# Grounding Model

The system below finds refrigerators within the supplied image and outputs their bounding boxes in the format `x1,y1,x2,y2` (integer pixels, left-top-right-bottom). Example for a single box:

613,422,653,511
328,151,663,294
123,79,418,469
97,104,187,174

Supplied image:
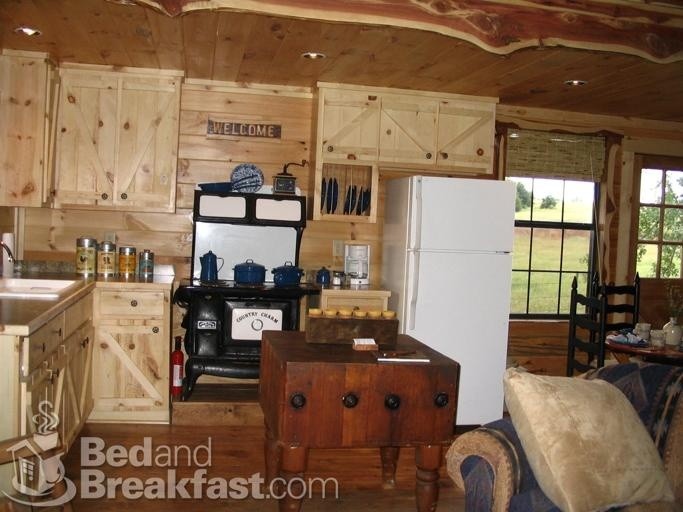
381,174,518,429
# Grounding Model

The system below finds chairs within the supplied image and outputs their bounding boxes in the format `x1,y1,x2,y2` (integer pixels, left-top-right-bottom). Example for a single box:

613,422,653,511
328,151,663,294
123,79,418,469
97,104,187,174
589,271,641,360
566,277,608,375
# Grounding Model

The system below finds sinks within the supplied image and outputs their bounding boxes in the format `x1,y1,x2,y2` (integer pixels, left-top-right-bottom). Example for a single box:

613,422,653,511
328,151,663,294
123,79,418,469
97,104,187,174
0,278,83,299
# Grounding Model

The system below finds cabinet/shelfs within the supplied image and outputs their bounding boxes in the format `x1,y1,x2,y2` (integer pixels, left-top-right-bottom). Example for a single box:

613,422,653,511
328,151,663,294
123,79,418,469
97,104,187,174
308,79,500,221
53,57,183,215
88,278,172,427
0,294,94,484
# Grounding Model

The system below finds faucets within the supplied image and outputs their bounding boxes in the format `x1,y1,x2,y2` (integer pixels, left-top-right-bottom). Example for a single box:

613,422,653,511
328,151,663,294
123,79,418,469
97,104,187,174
0,241,15,264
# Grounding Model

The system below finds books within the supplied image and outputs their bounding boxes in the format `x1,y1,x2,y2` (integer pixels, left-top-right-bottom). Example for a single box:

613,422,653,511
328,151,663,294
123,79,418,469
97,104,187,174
368,348,431,363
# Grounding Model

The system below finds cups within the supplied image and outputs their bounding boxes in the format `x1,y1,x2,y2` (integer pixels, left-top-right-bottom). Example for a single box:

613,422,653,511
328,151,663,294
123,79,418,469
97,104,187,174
649,330,667,348
634,322,652,339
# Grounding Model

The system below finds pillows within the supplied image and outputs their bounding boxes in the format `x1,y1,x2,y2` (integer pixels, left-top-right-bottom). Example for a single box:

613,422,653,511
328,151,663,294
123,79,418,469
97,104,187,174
504,366,674,512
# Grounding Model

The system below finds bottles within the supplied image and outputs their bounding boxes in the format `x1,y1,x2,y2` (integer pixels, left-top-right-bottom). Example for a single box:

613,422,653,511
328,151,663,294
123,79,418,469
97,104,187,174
663,316,681,346
74,237,96,282
117,246,135,283
168,335,183,397
96,241,115,282
139,248,153,283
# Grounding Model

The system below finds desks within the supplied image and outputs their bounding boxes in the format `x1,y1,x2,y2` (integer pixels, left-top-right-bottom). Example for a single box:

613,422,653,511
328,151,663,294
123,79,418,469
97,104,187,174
259,329,460,512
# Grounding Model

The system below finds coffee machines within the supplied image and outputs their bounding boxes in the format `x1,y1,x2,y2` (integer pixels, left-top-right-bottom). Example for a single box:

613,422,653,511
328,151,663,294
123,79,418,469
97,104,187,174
343,238,370,285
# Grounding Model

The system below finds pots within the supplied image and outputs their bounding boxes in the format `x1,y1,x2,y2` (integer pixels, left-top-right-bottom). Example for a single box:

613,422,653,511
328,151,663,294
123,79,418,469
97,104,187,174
197,183,233,194
272,260,305,286
232,258,266,286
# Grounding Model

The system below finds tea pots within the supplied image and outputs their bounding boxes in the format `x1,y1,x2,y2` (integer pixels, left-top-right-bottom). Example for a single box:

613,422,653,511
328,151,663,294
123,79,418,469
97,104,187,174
316,266,330,284
199,249,224,287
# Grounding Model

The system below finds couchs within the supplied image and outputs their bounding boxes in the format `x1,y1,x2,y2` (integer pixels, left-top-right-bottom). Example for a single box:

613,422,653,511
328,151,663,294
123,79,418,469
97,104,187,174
447,361,683,512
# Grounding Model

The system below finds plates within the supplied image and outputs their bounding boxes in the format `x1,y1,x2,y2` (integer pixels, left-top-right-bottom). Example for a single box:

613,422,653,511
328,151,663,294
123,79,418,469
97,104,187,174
229,164,264,194
317,177,370,215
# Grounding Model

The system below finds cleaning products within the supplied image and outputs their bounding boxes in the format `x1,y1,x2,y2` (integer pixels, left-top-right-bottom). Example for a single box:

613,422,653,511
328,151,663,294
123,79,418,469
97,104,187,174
2,232,14,265
